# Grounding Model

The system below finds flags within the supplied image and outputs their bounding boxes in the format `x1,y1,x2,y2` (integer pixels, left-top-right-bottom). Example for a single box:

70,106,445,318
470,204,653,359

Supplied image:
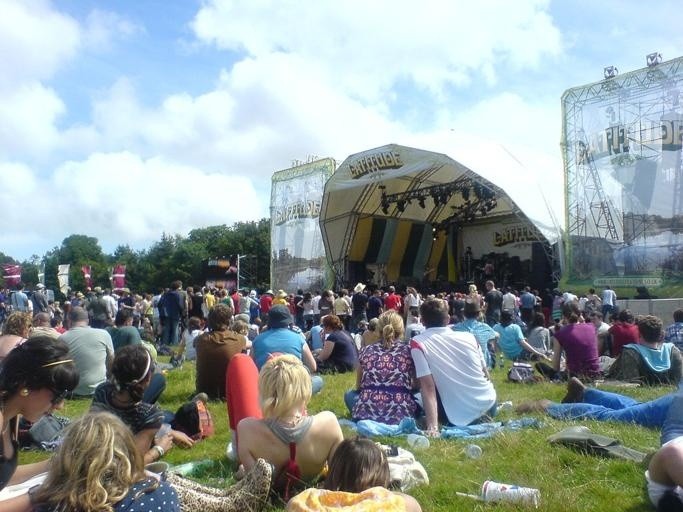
37,263,125,295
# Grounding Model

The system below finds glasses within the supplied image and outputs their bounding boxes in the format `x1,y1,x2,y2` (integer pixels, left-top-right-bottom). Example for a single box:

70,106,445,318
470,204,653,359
49,386,72,404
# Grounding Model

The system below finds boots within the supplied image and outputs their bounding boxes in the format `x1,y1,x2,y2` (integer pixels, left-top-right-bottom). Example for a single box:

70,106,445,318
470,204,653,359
160,458,274,512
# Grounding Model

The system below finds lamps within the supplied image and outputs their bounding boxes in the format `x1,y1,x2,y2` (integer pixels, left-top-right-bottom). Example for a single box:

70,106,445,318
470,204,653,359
430,194,499,241
646,52,663,66
378,175,498,216
602,65,619,79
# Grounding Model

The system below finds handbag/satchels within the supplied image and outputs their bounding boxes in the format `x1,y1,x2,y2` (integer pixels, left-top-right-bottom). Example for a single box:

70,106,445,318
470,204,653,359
377,439,429,491
510,363,532,381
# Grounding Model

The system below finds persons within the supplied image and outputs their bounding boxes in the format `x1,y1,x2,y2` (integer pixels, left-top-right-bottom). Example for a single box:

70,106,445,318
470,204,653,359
482,261,494,279
0,280,683,512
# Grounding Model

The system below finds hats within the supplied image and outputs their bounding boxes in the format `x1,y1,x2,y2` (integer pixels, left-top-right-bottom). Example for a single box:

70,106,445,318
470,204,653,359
354,283,365,293
268,305,292,326
266,290,273,294
277,290,287,298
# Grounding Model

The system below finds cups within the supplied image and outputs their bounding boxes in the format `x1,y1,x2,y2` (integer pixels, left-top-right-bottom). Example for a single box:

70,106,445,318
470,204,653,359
144,460,169,475
405,432,430,453
480,480,540,509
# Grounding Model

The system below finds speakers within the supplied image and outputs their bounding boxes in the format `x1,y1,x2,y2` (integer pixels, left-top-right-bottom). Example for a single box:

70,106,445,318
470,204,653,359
532,242,552,290
348,260,366,291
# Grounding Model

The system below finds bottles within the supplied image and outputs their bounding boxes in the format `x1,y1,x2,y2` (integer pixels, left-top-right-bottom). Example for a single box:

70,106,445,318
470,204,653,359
465,442,483,459
152,423,171,443
